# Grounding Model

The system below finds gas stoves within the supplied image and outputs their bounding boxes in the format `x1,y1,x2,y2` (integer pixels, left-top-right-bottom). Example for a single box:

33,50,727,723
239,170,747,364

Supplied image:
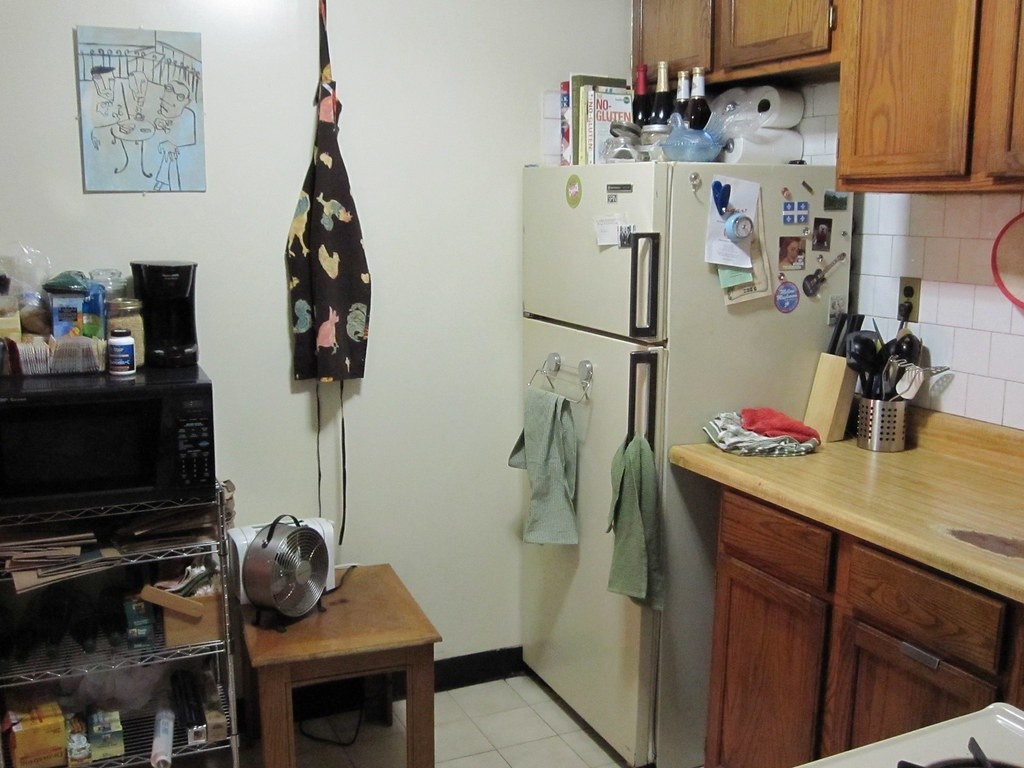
793,701,1024,768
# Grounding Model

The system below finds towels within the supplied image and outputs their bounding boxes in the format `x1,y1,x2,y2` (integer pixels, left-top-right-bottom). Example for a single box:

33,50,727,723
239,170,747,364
505,384,579,546
606,432,663,604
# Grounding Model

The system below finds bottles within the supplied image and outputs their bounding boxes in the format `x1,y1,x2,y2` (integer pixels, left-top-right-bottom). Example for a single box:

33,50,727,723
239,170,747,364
641,125,670,145
631,60,711,131
107,330,136,375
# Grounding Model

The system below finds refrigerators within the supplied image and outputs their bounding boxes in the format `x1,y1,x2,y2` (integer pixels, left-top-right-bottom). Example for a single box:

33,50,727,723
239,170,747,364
519,162,854,768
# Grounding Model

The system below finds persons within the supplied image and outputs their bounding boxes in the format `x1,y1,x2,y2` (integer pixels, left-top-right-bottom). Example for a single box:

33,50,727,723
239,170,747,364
780,237,802,269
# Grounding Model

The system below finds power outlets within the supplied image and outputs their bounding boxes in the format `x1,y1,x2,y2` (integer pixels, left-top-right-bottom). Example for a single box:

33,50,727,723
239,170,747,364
896,276,923,322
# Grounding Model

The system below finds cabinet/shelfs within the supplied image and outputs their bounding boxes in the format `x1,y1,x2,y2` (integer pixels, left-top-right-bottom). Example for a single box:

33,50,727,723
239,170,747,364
0,480,248,768
633,0,1024,195
698,487,1024,768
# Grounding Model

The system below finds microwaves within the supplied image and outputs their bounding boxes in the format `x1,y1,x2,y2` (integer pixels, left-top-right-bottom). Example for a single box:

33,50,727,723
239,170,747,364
0,362,217,516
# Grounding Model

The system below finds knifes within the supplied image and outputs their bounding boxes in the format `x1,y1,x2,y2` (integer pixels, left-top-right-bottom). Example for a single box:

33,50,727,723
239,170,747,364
827,313,865,356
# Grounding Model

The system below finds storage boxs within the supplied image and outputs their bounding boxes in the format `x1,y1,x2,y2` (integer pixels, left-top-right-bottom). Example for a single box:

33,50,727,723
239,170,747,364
165,592,223,646
7,693,68,768
87,709,126,761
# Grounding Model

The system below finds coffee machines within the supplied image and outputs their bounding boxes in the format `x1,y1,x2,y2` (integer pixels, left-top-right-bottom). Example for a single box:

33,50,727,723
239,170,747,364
129,260,199,368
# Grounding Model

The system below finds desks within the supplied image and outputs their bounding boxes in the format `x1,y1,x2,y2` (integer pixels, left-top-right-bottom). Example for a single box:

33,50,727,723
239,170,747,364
240,561,445,768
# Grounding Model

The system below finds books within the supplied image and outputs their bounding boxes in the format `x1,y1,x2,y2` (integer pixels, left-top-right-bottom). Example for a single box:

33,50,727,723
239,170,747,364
1,505,218,594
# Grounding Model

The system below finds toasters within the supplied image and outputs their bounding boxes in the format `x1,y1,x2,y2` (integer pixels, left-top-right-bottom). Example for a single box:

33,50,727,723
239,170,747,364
227,517,335,607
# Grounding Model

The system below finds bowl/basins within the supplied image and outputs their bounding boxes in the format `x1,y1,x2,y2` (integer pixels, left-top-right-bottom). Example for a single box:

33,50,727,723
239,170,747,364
661,142,722,162
